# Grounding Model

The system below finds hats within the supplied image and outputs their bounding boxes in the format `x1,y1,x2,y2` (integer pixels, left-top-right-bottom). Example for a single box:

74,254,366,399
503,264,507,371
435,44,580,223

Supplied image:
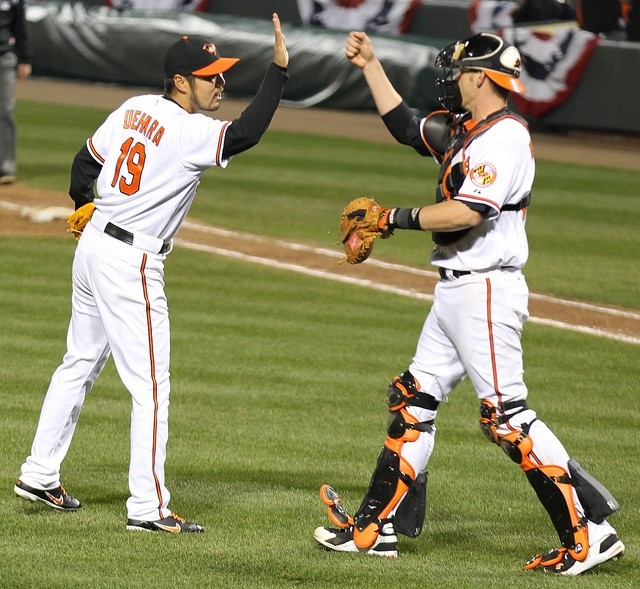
165,35,239,77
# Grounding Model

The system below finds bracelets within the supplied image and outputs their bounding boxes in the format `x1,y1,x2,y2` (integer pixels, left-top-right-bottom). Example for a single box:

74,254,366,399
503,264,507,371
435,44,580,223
388,207,424,231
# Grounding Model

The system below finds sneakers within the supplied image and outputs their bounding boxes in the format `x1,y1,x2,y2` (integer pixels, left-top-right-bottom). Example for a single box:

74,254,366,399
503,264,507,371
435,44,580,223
126,513,204,533
314,520,397,559
14,478,82,511
0,170,17,184
542,520,624,575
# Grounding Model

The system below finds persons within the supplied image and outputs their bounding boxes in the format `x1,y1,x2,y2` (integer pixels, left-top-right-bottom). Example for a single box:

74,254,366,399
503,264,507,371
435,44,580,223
312,32,625,577
15,13,290,536
1,1,31,185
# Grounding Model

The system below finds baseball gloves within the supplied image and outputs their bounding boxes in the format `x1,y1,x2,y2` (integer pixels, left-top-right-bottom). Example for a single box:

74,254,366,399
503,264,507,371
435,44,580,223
339,196,398,267
65,201,97,238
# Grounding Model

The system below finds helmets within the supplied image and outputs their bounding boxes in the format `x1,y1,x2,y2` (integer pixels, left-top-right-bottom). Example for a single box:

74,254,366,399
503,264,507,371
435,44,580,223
435,32,527,127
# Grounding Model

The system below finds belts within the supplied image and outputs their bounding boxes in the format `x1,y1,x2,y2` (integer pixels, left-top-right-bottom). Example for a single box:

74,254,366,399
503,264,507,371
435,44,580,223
89,209,172,255
438,266,515,280
